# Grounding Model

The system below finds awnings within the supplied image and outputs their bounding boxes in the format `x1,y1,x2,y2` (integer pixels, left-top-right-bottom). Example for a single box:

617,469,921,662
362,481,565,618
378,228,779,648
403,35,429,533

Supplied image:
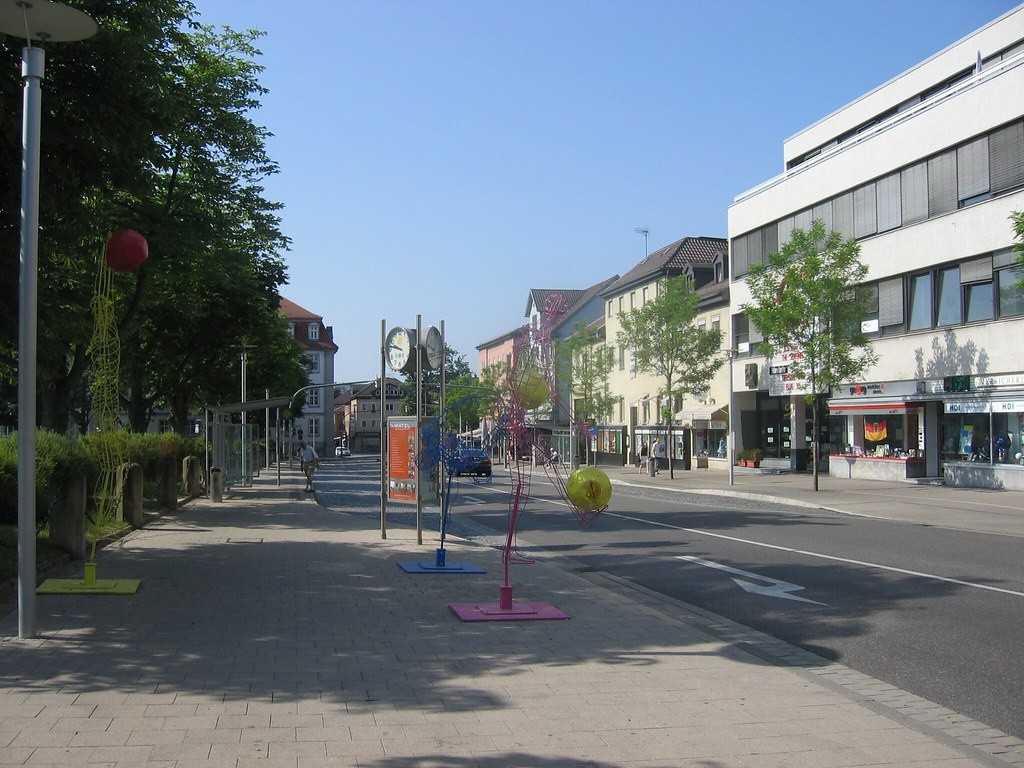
675,403,728,421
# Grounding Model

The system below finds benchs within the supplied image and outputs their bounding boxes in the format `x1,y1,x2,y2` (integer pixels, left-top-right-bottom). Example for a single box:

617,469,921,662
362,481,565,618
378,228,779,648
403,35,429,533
544,454,561,466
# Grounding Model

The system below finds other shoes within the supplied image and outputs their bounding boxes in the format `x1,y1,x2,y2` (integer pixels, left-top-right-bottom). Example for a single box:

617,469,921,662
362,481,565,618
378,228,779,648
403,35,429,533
303,489,314,493
546,465,551,469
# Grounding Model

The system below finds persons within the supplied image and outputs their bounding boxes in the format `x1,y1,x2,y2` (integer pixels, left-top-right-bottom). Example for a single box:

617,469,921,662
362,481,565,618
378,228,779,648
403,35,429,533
639,441,648,474
716,437,725,458
652,438,665,474
299,440,319,493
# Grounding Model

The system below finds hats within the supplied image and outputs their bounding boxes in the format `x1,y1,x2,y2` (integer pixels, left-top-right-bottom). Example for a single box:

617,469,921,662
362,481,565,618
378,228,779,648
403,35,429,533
550,448,554,451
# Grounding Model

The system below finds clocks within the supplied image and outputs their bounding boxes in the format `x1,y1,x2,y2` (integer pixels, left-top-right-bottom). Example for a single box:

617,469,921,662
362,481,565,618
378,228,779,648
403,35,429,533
422,325,443,372
385,327,417,372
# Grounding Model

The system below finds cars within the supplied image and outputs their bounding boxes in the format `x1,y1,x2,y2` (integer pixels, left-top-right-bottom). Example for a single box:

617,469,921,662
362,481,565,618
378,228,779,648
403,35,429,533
445,449,492,477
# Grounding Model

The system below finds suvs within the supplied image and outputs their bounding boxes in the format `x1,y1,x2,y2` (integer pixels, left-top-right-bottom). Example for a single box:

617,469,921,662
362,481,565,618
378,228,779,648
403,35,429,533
336,447,351,456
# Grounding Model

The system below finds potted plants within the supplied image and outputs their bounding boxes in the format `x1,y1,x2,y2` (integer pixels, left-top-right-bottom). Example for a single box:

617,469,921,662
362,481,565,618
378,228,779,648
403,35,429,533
735,449,765,468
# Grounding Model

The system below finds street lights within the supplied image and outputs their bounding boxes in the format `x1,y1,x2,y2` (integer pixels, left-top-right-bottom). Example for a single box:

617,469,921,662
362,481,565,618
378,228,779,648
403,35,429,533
265,385,269,473
232,344,258,486
2,3,97,638
714,349,744,486
